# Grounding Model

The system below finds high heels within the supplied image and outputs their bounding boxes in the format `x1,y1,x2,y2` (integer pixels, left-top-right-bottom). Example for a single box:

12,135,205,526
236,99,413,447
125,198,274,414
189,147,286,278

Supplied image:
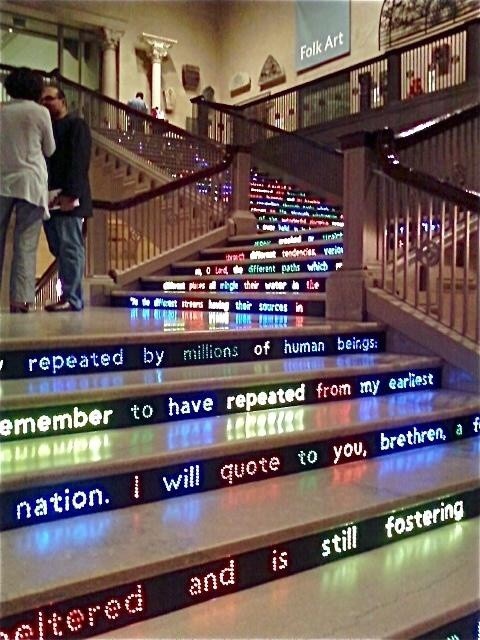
10,301,29,313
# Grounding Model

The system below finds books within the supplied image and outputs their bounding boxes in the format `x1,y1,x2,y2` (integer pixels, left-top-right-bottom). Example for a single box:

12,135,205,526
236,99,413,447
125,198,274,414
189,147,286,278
48,188,79,209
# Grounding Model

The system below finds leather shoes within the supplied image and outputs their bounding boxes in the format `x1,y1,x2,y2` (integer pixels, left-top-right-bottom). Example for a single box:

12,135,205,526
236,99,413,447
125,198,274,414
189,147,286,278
45,299,75,311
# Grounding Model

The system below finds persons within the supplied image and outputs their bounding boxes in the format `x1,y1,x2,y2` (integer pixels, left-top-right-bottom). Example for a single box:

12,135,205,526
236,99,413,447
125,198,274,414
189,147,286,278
40,86,93,311
126,92,148,133
274,113,282,131
1,66,55,313
